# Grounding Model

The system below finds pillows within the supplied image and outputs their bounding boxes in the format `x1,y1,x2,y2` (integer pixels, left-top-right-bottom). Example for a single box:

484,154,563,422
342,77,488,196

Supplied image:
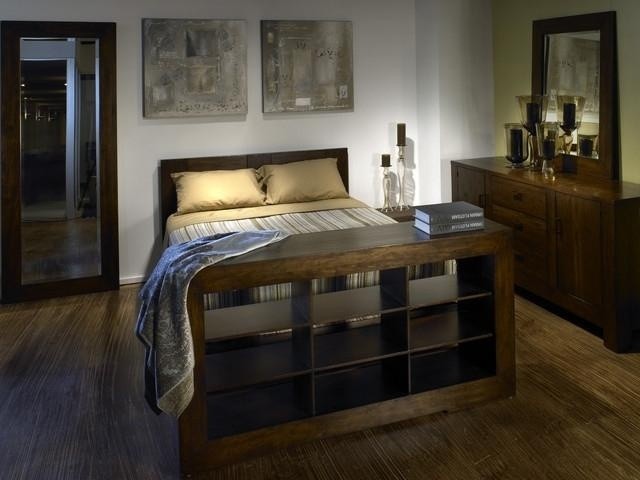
256,158,350,205
169,167,267,214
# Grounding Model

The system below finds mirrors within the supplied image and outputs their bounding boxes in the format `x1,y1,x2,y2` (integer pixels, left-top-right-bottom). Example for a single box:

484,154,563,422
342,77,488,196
531,12,617,180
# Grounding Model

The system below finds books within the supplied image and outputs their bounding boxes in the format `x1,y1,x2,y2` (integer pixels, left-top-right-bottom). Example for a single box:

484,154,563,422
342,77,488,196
412,201,483,224
412,217,484,236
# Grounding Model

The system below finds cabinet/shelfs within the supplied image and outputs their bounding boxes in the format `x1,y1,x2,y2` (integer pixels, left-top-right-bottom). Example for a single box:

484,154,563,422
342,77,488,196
451,155,640,354
178,217,515,474
636,153,640,156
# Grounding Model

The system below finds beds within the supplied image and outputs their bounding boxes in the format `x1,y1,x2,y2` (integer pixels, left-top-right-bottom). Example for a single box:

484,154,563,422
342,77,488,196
159,147,457,336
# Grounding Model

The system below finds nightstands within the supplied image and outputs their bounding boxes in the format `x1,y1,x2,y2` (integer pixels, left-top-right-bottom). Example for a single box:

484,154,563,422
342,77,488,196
376,206,415,222
